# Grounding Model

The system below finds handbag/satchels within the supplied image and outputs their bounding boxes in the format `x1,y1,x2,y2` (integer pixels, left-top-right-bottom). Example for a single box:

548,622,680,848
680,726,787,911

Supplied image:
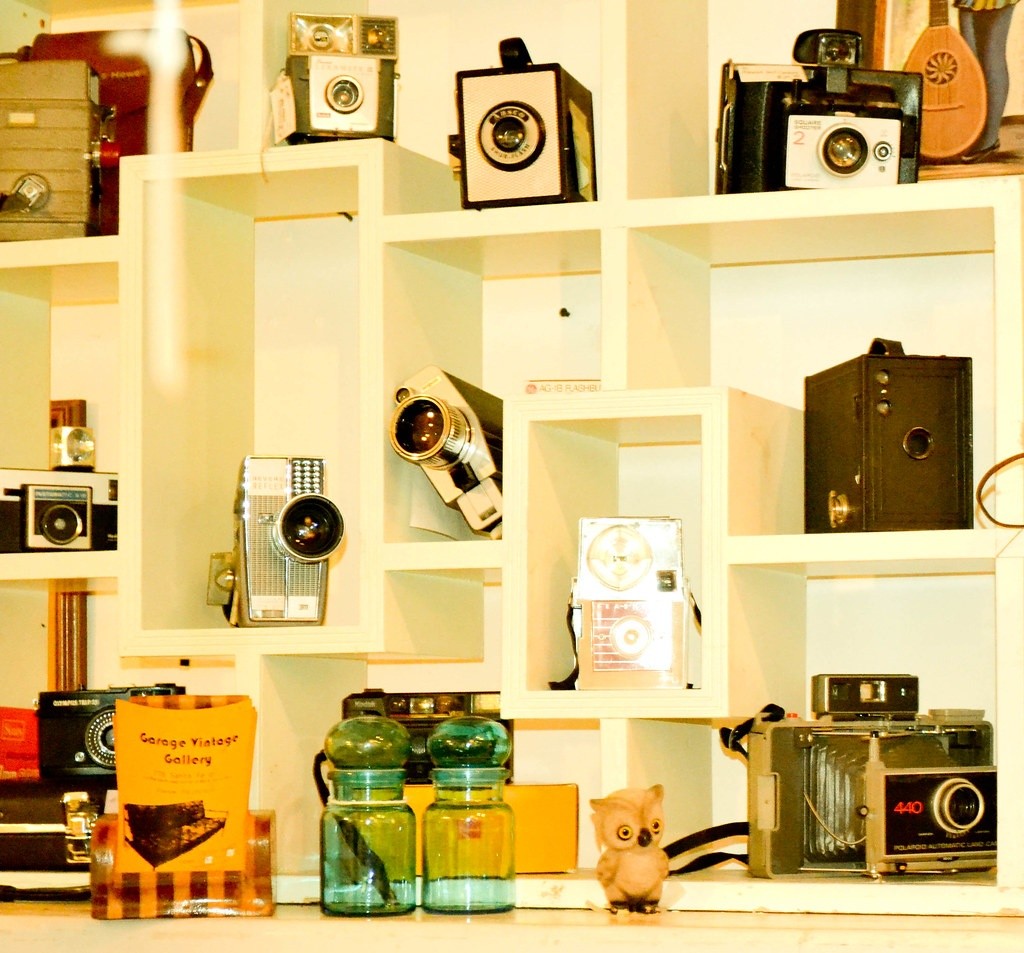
2,28,213,239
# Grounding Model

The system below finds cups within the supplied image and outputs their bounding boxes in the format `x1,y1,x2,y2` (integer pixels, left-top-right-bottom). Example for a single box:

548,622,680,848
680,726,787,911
421,716,517,915
317,716,416,913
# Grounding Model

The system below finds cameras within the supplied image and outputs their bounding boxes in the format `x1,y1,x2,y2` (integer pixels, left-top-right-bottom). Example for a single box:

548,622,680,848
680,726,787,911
805,355,975,533
569,516,692,691
716,27,923,195
387,363,504,540
2,59,122,242
449,37,597,209
0,425,120,553
284,11,400,143
748,673,999,880
216,455,343,627
342,688,514,784
36,683,186,781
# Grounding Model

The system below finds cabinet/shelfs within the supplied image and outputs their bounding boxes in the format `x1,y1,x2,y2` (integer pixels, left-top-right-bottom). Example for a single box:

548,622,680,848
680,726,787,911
0,0,1024,915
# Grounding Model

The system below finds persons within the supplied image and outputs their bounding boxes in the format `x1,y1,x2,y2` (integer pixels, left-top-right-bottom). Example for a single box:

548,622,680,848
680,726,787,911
952,0,1020,163
